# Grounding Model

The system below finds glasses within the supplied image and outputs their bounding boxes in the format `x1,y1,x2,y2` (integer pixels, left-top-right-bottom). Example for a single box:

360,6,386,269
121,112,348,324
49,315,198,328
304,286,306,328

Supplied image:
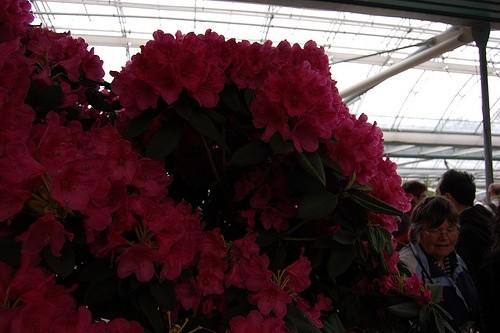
422,226,459,237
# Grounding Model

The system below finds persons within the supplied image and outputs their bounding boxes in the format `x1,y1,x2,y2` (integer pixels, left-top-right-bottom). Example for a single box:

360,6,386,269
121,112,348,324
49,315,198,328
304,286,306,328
394,195,475,333
434,170,500,333
483,182,500,216
401,180,427,215
